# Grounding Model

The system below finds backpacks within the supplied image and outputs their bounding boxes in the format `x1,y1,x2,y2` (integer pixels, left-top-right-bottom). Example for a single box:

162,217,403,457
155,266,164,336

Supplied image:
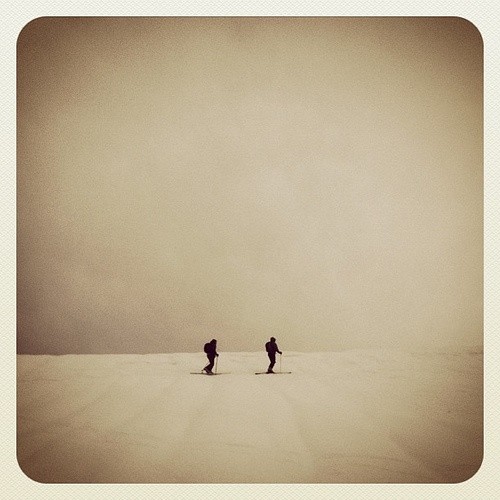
203,342,210,352
266,342,275,354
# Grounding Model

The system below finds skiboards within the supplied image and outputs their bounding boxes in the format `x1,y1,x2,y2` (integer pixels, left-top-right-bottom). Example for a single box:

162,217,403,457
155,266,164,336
190,372,222,375
254,372,292,374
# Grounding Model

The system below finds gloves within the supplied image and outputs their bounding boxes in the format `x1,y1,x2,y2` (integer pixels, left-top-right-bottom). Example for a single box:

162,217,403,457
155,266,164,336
280,352,282,354
216,353,218,356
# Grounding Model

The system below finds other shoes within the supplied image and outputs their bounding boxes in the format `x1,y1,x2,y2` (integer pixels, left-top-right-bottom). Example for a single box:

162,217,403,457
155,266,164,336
268,369,274,373
204,367,211,374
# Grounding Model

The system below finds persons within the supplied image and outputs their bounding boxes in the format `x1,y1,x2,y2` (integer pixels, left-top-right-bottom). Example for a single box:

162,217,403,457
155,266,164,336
204,339,219,374
265,337,282,373
